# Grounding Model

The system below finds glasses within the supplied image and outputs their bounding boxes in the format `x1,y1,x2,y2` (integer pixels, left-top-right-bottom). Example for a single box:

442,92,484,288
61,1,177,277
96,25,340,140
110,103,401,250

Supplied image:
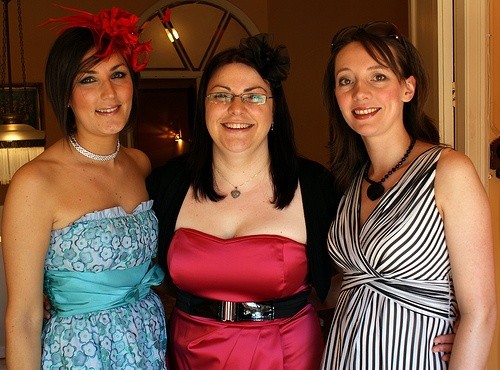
204,92,272,106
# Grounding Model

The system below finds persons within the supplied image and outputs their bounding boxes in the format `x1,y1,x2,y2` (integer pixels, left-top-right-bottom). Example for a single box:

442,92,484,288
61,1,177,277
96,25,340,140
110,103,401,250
146,47,461,370
2,26,168,370
321,20,497,370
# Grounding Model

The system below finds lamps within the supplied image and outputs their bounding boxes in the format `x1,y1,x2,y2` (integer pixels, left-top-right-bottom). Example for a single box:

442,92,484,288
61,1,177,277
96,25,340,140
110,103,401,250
0,0,47,185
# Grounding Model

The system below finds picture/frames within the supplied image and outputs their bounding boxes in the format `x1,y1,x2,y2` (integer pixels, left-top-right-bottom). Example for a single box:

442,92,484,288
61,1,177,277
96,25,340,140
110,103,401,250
0,83,44,132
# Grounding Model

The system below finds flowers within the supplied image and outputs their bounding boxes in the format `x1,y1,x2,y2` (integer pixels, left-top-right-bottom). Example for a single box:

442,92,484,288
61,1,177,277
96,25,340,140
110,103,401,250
238,32,291,82
33,2,175,73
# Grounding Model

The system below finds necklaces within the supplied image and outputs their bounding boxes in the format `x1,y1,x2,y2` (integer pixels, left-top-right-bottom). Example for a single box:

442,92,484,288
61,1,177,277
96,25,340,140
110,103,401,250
216,161,268,198
365,136,416,201
69,133,120,161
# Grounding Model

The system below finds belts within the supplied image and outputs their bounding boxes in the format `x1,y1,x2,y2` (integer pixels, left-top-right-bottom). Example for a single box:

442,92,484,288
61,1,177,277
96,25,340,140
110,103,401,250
174,286,312,323
